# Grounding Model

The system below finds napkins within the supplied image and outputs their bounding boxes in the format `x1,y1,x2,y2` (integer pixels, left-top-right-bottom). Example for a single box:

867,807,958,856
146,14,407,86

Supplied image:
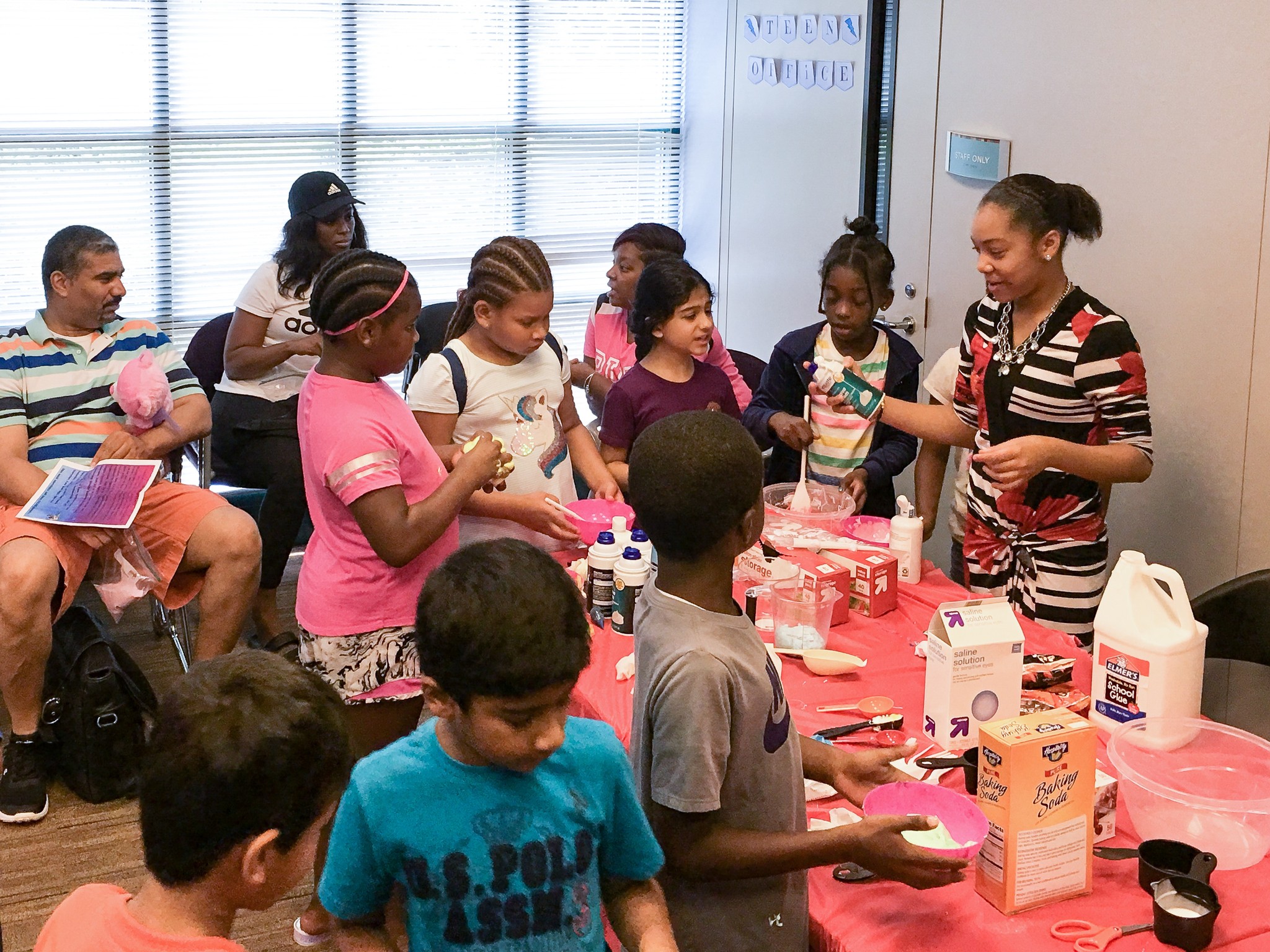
808,808,863,831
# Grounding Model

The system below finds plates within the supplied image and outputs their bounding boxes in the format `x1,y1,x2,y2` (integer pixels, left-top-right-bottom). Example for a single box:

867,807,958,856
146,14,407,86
842,515,891,548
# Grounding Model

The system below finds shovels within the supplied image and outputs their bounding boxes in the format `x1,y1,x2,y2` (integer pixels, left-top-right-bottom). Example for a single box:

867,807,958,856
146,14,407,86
789,396,814,513
774,646,868,675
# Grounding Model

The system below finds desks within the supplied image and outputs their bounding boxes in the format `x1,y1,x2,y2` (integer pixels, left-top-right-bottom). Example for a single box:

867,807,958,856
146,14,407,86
546,547,1270,952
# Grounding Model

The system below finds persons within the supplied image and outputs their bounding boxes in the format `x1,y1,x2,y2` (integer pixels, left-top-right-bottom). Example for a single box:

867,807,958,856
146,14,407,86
0,224,264,822
33,649,357,951
582,222,753,451
743,215,925,518
404,235,624,553
319,538,679,952
803,174,1154,653
293,250,513,946
627,410,970,952
598,259,742,491
209,171,369,665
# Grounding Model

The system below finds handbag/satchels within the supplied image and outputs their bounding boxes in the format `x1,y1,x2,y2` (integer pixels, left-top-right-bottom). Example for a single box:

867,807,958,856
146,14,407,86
42,605,159,801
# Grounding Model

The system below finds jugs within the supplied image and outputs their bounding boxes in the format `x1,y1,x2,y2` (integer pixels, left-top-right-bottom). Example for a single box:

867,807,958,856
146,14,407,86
732,557,801,632
744,579,844,659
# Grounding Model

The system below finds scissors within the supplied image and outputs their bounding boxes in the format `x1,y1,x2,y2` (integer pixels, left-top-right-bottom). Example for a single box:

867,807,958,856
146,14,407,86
1052,919,1155,952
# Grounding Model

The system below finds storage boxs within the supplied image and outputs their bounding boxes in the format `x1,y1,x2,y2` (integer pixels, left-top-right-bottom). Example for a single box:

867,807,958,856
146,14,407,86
818,548,898,618
923,595,1025,751
974,708,1098,916
1093,769,1119,845
734,536,851,624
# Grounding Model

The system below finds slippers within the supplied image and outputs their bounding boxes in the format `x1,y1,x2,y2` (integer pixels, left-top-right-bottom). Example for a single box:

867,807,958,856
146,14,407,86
247,630,299,664
293,917,332,947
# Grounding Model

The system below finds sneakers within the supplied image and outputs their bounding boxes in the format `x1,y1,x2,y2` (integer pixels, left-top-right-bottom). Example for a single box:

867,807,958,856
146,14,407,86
0,735,50,825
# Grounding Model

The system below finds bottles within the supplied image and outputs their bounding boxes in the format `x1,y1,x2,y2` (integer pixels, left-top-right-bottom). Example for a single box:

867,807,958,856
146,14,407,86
585,531,624,619
889,495,923,584
611,546,650,636
623,529,652,565
807,362,885,420
606,516,633,552
1088,550,1209,753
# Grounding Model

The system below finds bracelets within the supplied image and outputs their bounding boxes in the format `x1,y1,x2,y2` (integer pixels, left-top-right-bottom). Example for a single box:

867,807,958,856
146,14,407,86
876,400,885,421
584,373,594,393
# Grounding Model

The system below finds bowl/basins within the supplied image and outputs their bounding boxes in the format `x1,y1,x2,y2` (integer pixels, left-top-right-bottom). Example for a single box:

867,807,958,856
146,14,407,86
763,482,856,536
563,499,636,546
863,781,989,861
1106,716,1270,870
1138,839,1210,897
1150,875,1222,952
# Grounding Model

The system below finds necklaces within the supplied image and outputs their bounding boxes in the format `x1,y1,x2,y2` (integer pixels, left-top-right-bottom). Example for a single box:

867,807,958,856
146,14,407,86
990,283,1071,376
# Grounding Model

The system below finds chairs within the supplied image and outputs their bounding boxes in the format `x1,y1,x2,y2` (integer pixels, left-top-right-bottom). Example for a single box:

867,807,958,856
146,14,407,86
183,312,265,518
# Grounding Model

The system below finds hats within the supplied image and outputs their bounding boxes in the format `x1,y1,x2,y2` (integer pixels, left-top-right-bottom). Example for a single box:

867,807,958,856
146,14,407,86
289,171,365,219
